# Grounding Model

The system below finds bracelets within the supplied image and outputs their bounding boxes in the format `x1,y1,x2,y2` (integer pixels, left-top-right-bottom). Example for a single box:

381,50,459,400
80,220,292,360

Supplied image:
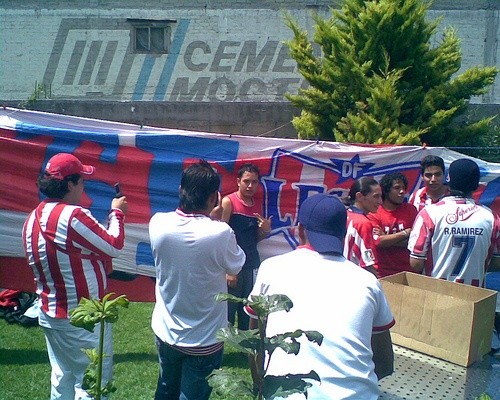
402,230,409,238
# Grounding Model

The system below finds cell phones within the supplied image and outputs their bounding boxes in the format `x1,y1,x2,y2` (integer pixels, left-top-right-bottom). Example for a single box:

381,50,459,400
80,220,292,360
114,183,122,198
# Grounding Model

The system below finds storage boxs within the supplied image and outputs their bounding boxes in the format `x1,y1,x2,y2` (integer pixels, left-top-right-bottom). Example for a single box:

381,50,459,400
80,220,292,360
377,271,498,368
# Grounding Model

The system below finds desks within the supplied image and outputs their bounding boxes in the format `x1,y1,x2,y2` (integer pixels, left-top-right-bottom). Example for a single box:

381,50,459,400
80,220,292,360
378,343,500,400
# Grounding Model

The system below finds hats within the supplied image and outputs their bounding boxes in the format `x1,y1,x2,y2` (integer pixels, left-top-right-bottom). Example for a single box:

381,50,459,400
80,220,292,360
446,158,480,191
297,193,347,256
45,153,96,179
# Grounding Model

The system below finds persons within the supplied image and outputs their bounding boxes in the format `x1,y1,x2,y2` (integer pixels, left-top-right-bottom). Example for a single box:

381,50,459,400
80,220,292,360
408,155,450,212
407,158,500,289
148,160,247,400
368,172,418,276
244,194,395,400
222,164,273,337
343,177,383,278
22,154,129,400
